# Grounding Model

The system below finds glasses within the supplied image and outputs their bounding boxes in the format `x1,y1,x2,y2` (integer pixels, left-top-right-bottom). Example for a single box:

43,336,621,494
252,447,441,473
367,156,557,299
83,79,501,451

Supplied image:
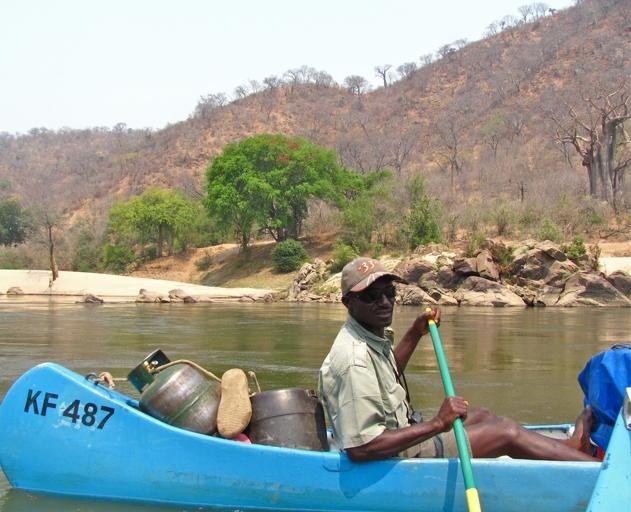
352,285,397,303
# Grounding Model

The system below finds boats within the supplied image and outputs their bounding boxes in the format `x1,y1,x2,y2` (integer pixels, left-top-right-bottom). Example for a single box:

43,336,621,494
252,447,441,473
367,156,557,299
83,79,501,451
0,362,631,512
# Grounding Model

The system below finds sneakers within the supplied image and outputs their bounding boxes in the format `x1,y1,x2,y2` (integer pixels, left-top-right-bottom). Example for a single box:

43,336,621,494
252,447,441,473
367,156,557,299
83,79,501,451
216,368,252,438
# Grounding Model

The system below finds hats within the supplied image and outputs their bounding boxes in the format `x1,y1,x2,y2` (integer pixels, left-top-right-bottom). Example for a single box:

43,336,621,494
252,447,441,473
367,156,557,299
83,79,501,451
341,258,409,296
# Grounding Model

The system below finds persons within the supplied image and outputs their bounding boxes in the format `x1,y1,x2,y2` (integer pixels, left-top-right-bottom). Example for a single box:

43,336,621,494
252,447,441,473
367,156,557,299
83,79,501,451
316,256,602,461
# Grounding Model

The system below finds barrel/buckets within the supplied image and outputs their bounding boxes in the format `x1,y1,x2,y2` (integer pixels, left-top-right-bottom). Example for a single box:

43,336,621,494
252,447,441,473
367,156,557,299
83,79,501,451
242,387,327,451
